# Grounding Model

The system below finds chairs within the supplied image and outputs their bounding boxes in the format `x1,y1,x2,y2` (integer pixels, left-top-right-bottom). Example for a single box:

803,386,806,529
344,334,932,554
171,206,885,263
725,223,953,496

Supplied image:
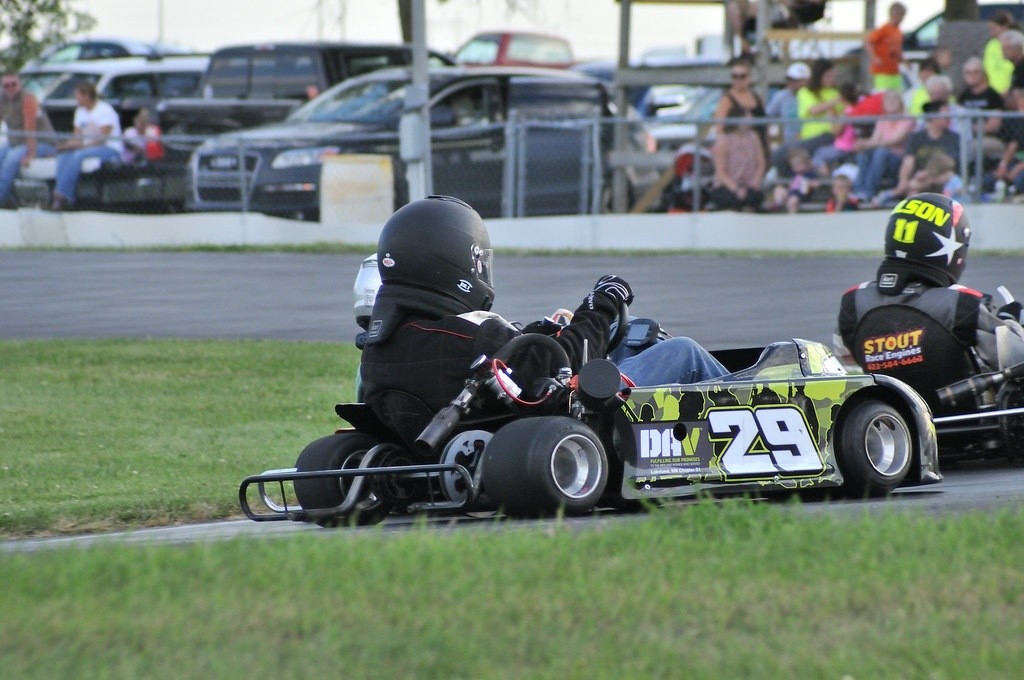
852,304,997,427
371,390,519,461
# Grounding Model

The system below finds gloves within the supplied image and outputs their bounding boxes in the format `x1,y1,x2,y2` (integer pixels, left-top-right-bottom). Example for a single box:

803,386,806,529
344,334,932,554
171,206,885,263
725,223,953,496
523,319,563,338
997,301,1022,320
595,273,634,309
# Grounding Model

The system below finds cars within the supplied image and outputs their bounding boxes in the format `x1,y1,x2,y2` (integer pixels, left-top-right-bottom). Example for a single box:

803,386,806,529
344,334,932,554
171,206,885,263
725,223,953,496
0,0,1024,214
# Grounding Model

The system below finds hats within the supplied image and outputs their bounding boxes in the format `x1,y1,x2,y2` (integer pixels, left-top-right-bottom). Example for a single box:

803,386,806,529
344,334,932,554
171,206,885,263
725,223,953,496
786,61,813,80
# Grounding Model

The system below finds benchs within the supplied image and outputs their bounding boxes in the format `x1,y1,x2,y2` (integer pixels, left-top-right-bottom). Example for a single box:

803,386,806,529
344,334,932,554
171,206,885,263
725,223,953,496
18,157,167,214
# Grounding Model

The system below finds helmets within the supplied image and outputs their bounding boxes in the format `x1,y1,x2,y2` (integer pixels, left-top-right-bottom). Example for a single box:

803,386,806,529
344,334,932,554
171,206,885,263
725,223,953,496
376,194,495,308
353,255,384,328
884,191,972,282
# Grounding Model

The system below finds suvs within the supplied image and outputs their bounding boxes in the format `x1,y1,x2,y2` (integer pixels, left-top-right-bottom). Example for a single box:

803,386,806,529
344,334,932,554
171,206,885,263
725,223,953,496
182,63,657,223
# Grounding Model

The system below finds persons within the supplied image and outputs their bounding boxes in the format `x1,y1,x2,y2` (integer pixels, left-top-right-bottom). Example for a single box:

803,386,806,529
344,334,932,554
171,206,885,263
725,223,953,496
52,82,122,208
839,193,1024,417
360,195,730,419
991,10,1024,110
112,112,147,177
0,71,56,207
722,0,826,63
865,2,907,89
712,58,1024,212
